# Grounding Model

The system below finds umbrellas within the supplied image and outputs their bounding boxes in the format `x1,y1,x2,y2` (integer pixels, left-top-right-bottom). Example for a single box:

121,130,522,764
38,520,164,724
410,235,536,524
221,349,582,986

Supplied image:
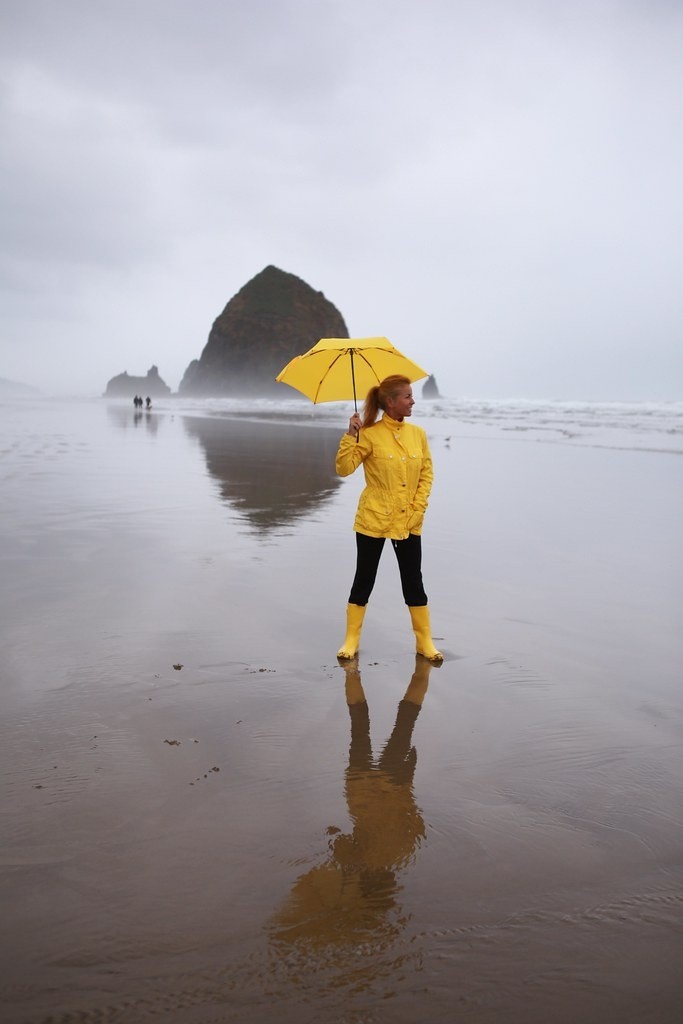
274,336,430,444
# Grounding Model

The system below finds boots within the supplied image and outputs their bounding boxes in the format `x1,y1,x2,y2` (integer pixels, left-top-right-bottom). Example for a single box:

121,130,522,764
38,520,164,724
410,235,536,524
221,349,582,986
337,603,368,660
338,654,366,706
403,654,442,706
409,605,444,662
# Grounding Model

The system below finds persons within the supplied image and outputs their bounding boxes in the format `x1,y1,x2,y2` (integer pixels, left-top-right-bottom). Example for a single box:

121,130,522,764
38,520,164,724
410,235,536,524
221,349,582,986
134,395,151,408
335,373,445,663
